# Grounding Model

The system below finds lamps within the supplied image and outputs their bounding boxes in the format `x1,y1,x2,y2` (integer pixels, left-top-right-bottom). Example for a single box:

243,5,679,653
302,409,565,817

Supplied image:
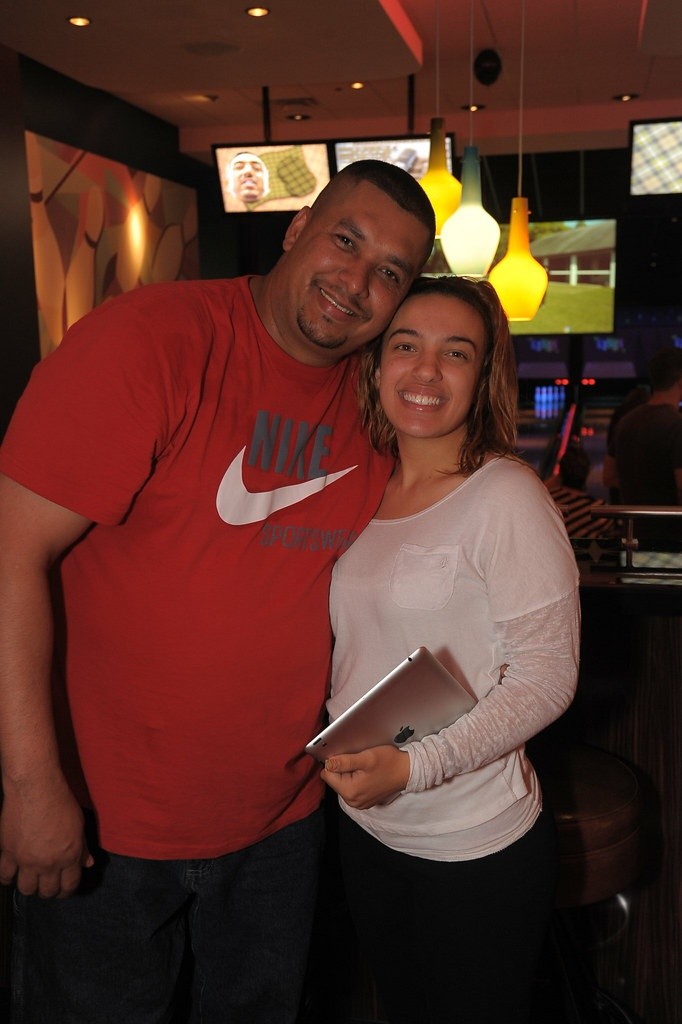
439,0,500,277
487,0,548,323
416,0,465,241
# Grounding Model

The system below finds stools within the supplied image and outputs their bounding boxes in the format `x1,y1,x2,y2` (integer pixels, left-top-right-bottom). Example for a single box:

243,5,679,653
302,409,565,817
534,750,645,1024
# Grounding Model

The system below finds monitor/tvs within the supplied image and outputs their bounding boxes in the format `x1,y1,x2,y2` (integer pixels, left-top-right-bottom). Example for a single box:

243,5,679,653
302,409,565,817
624,118,682,199
211,132,455,217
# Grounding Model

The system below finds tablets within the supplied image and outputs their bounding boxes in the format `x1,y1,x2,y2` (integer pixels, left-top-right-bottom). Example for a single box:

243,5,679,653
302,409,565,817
304,647,477,761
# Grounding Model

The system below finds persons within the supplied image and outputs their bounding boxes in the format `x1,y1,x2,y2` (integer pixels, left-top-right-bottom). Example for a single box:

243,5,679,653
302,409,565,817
228,153,269,202
0,160,436,1024
313,276,581,1024
586,346,682,555
